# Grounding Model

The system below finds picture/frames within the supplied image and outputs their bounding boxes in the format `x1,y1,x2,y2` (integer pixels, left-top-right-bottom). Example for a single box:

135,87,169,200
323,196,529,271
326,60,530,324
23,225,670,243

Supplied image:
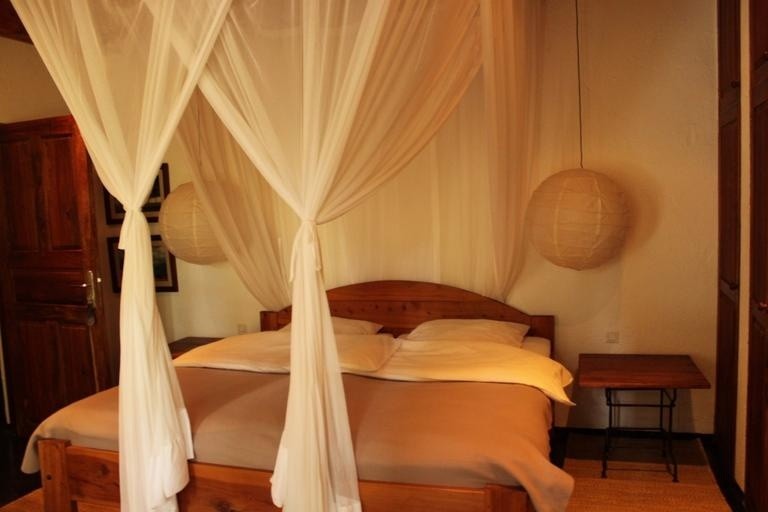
104,162,172,225
106,234,179,293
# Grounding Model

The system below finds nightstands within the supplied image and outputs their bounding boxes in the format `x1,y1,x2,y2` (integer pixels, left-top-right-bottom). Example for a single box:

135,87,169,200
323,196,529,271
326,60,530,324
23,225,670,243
162,336,226,359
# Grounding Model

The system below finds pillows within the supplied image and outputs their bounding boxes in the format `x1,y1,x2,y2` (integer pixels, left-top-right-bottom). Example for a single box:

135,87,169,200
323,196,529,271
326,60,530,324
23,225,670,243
408,319,531,349
277,315,383,334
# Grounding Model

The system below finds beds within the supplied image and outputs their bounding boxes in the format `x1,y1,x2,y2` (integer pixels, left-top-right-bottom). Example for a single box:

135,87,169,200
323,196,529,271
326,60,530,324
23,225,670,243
22,278,575,510
